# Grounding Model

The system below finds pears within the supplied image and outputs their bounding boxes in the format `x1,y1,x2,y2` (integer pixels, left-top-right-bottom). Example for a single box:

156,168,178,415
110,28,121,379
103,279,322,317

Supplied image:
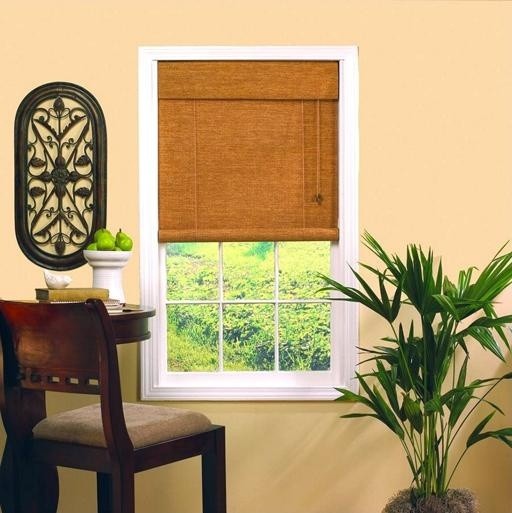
87,226,132,251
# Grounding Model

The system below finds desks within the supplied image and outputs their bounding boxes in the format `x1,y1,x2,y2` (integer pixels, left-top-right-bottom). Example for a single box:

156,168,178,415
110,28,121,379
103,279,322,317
35,287,157,345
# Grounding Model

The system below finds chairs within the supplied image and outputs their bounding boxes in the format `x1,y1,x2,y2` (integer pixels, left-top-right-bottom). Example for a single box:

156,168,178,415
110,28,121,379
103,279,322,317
0,297,227,512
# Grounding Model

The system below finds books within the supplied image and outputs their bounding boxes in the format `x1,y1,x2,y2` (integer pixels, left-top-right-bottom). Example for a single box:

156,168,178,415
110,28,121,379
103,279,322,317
34,286,125,314
